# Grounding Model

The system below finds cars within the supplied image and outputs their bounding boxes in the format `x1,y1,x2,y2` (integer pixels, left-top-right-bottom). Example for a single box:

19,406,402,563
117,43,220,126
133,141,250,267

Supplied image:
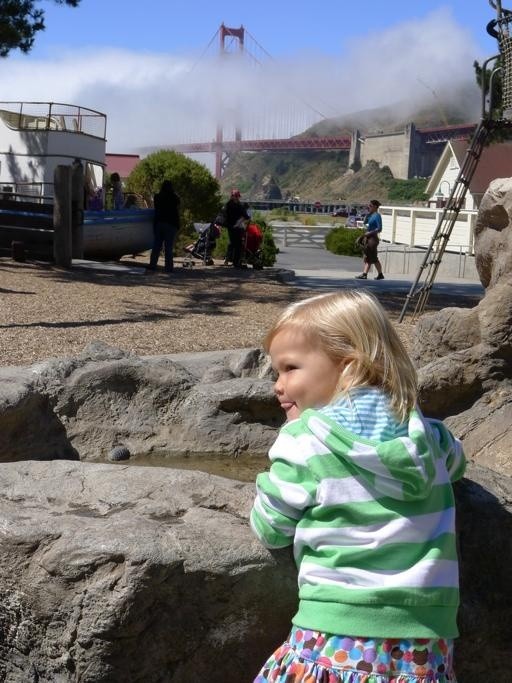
333,208,357,218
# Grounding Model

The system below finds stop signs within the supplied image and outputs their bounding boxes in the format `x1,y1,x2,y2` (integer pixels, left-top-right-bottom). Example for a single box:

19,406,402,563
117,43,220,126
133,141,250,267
314,201,322,209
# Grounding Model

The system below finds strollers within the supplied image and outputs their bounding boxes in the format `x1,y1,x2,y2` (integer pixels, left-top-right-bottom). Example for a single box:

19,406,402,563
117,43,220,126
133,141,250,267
240,220,265,268
182,219,220,267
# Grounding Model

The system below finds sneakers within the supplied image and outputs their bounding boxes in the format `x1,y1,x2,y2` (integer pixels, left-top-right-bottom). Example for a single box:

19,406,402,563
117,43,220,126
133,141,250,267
374,273,385,280
354,273,368,279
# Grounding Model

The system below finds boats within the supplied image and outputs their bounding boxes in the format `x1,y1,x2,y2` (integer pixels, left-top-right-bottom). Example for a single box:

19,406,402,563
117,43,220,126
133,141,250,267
0,181,156,262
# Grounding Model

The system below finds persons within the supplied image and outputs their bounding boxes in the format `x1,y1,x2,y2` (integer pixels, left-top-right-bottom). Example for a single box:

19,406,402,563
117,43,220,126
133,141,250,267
110,173,124,209
217,188,252,269
250,289,468,683
125,196,140,208
145,180,181,275
355,199,385,280
